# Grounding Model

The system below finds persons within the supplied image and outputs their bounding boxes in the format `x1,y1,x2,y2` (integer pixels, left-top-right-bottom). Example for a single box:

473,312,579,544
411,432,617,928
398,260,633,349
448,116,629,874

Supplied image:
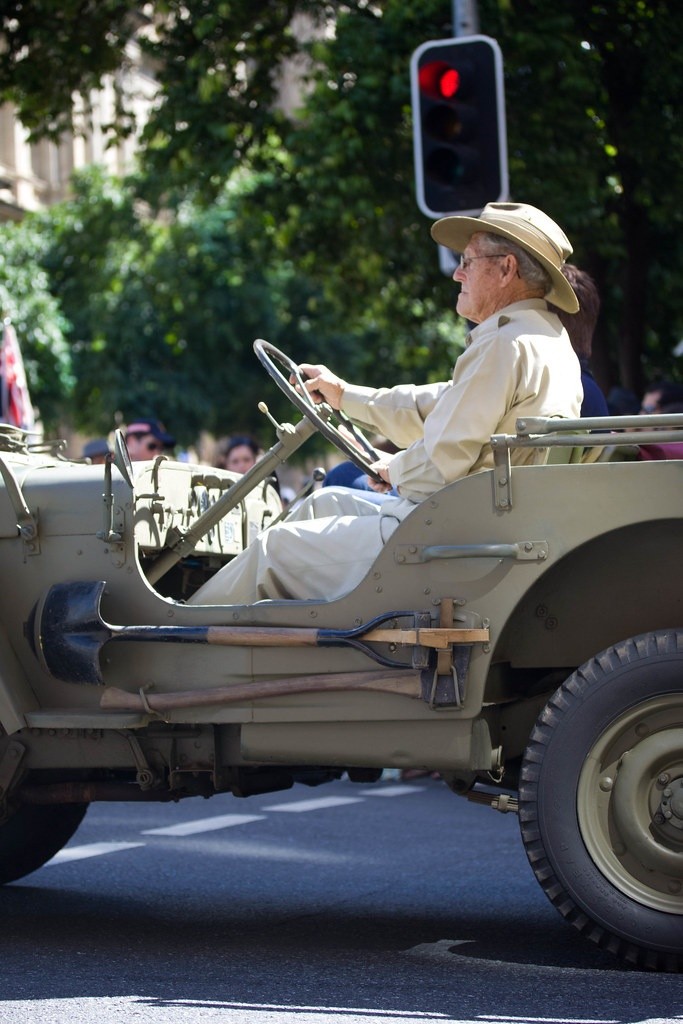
180,200,586,600
321,260,611,485
83,409,174,461
217,439,258,476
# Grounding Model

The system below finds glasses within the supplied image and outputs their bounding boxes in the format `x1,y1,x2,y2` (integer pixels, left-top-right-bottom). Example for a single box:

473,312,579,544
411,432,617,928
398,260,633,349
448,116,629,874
460,254,522,280
148,442,158,450
641,405,657,413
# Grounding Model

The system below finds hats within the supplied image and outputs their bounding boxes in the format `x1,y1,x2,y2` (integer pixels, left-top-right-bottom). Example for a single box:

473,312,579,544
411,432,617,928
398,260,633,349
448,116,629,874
431,202,580,314
80,440,107,458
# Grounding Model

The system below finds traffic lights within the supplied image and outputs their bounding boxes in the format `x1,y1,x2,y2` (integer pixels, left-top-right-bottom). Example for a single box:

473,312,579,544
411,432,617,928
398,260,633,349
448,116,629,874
409,32,510,219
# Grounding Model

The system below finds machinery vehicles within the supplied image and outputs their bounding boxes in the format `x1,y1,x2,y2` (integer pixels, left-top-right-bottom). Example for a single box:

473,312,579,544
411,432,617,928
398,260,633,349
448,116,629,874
0,340,683,977
